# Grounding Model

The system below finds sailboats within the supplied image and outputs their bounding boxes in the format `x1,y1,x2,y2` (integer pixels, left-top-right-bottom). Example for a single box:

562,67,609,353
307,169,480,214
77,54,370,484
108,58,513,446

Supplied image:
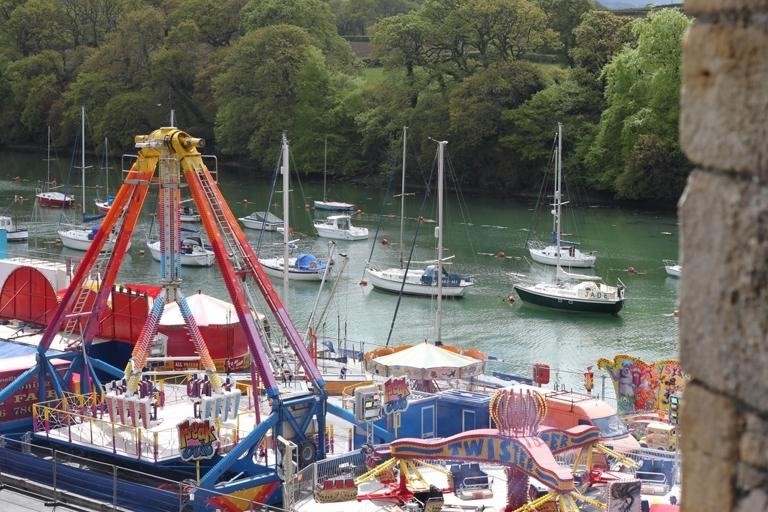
58,106,131,253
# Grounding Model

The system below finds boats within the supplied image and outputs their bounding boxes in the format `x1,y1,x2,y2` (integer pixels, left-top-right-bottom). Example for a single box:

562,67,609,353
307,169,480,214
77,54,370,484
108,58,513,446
179,202,201,222
59,230,131,252
7,227,29,242
663,258,685,279
314,215,370,241
146,240,215,267
238,217,284,230
313,200,355,212
312,137,355,213
179,209,202,223
239,212,287,230
313,219,369,240
662,259,680,278
146,235,216,267
0,216,28,241
